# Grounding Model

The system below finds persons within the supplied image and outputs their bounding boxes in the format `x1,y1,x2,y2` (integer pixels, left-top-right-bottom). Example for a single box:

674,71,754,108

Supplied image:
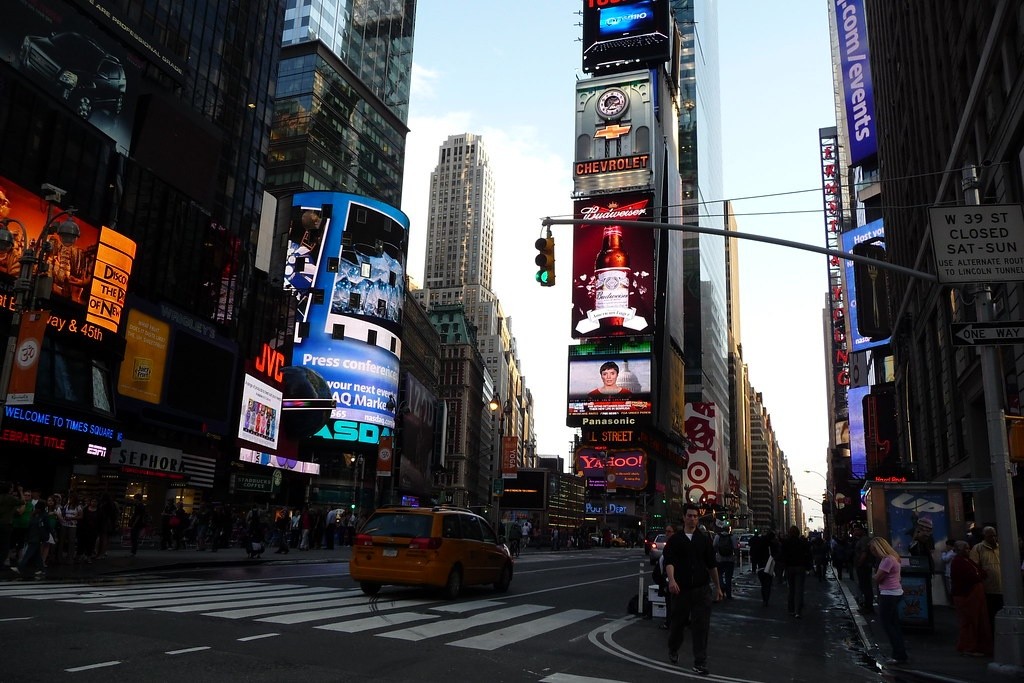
586,361,633,393
663,506,724,675
1,477,1004,666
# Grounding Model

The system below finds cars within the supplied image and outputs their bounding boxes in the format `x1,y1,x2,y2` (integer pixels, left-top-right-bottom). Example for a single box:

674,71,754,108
649,534,668,564
592,533,627,547
349,504,514,601
645,530,664,554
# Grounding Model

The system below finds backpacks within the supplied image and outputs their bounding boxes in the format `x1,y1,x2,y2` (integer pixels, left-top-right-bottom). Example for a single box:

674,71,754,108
717,531,734,558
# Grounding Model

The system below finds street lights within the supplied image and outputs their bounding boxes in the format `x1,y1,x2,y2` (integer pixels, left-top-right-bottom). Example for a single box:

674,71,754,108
0,207,81,424
806,470,827,482
384,394,411,503
490,393,513,479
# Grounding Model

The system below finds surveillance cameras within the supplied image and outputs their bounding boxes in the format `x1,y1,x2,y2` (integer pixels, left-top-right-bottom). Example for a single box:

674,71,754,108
42,184,67,196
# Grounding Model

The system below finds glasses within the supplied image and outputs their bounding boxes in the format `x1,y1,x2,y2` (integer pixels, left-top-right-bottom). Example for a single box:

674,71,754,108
24,493,31,497
985,534,997,538
664,530,673,533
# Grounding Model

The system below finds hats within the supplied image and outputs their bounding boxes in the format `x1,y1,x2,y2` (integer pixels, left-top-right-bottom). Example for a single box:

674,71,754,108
917,517,934,529
54,493,62,500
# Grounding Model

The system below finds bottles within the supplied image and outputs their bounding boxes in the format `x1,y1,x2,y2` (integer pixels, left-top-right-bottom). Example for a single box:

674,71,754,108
594,207,629,336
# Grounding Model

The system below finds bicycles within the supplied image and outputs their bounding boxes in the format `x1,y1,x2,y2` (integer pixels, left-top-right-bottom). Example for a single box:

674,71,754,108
510,538,520,557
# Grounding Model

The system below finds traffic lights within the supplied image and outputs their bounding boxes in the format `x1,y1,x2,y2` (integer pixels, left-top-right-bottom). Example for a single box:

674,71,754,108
535,235,556,287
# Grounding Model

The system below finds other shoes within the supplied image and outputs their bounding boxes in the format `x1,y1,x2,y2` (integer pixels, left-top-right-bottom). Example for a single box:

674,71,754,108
794,613,803,622
43,563,48,568
95,556,105,560
10,565,21,576
87,560,93,564
886,657,899,665
962,650,985,658
35,569,47,576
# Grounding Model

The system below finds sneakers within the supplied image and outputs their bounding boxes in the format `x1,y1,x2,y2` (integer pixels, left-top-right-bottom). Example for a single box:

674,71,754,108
691,664,711,676
668,649,680,665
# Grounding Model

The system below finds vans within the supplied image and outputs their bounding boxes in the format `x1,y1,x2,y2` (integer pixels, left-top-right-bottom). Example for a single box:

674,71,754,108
739,534,761,552
